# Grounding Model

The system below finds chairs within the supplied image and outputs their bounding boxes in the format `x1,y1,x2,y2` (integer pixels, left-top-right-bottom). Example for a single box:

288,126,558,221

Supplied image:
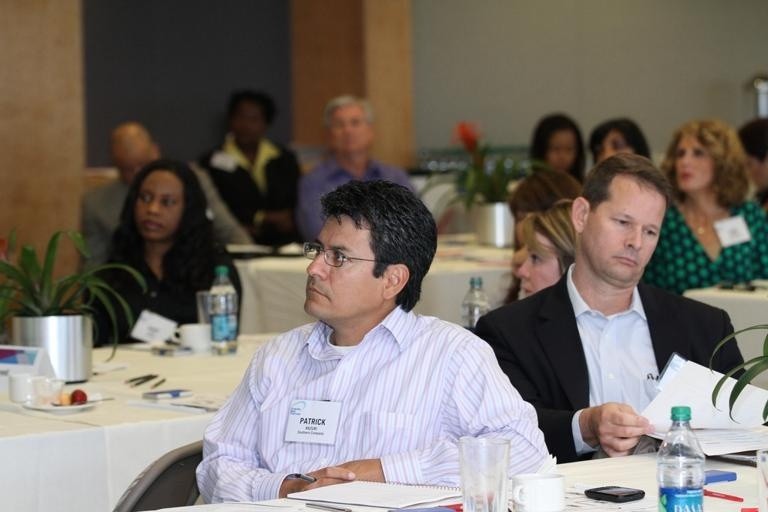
113,440,202,511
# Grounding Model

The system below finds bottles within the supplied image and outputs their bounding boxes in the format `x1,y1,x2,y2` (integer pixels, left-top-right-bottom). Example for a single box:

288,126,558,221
656,406,707,512
209,265,239,353
462,275,492,333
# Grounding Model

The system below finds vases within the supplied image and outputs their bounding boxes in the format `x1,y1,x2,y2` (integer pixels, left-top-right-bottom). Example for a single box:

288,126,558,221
472,190,519,248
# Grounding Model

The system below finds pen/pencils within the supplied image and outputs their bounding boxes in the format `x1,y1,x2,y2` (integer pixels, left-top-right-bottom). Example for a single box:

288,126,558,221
132,376,157,387
295,474,317,482
125,375,151,383
704,489,743,502
151,379,165,389
306,503,352,512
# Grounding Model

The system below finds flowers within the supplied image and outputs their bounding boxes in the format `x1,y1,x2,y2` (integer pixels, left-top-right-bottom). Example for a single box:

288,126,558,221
402,110,580,207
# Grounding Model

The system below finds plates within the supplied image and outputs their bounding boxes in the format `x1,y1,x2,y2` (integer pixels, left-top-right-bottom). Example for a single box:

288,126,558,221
23,392,103,415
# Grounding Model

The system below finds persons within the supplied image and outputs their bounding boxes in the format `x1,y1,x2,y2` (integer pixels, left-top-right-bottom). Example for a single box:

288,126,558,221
510,113,768,304
191,179,560,506
473,152,751,463
80,89,304,347
295,96,417,242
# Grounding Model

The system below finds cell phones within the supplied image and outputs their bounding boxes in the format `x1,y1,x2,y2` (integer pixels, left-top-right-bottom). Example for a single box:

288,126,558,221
585,486,644,502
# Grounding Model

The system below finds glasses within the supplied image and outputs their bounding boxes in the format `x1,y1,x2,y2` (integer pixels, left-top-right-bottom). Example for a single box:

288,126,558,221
303,242,392,267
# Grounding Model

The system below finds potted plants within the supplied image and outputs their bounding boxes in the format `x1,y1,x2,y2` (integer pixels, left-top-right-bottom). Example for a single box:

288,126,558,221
0,219,151,383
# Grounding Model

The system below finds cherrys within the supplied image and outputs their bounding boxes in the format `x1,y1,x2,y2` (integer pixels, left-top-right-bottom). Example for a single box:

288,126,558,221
70,389,87,404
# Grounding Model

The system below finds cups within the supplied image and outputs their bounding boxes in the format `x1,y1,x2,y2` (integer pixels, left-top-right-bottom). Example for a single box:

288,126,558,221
170,324,210,351
458,435,511,511
755,447,768,509
512,473,567,511
9,372,48,401
195,290,209,324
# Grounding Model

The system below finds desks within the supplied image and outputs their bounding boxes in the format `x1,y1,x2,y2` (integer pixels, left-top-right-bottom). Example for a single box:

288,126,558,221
680,276,768,386
145,446,766,512
0,330,285,512
232,231,518,345
